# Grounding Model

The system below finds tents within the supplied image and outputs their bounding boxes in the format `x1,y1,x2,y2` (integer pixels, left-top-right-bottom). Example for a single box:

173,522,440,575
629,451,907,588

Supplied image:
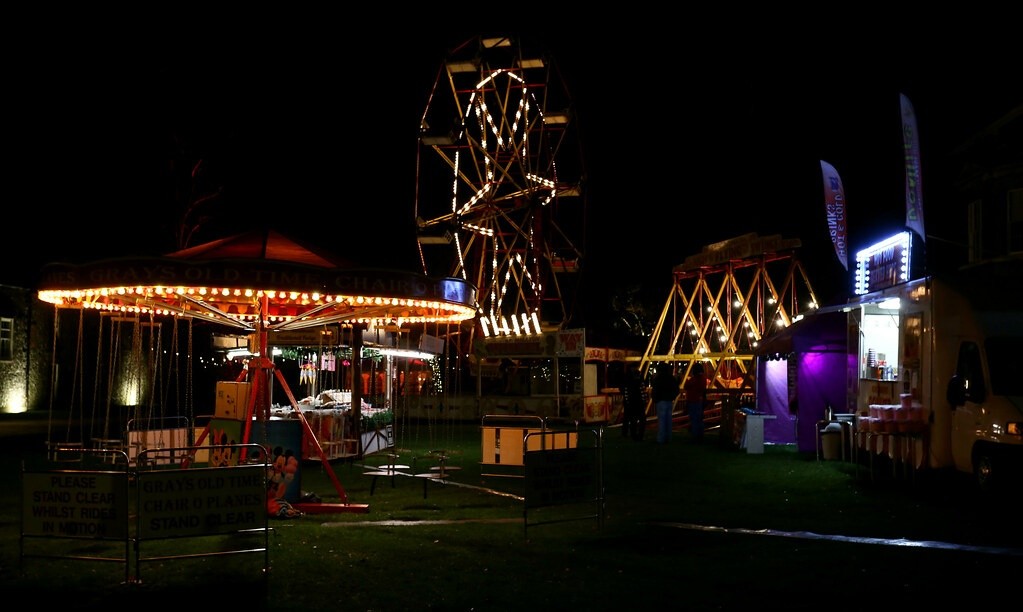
753,315,848,451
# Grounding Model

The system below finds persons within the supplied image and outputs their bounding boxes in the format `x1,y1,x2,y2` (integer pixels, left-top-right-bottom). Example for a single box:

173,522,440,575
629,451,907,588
651,363,679,443
618,361,647,443
684,363,706,439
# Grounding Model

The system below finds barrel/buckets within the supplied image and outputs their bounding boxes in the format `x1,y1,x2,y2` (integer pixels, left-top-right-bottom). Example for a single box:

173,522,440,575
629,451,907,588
820,430,840,459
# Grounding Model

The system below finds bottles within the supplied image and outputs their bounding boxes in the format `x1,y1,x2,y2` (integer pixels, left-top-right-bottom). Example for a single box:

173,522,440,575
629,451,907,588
888,365,893,379
883,366,887,379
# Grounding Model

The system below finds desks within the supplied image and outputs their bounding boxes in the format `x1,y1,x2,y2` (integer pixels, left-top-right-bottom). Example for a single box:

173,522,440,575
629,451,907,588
853,429,919,481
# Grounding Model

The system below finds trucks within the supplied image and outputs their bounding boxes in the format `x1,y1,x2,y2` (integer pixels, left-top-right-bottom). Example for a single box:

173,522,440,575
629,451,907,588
839,272,1023,486
